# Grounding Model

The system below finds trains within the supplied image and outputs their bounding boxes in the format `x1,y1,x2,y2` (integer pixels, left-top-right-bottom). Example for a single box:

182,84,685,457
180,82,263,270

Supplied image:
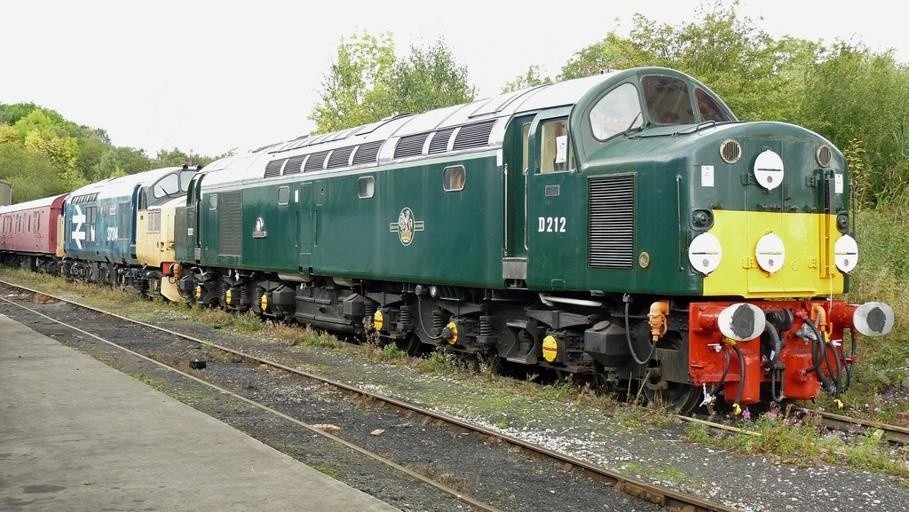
0,66,894,418
